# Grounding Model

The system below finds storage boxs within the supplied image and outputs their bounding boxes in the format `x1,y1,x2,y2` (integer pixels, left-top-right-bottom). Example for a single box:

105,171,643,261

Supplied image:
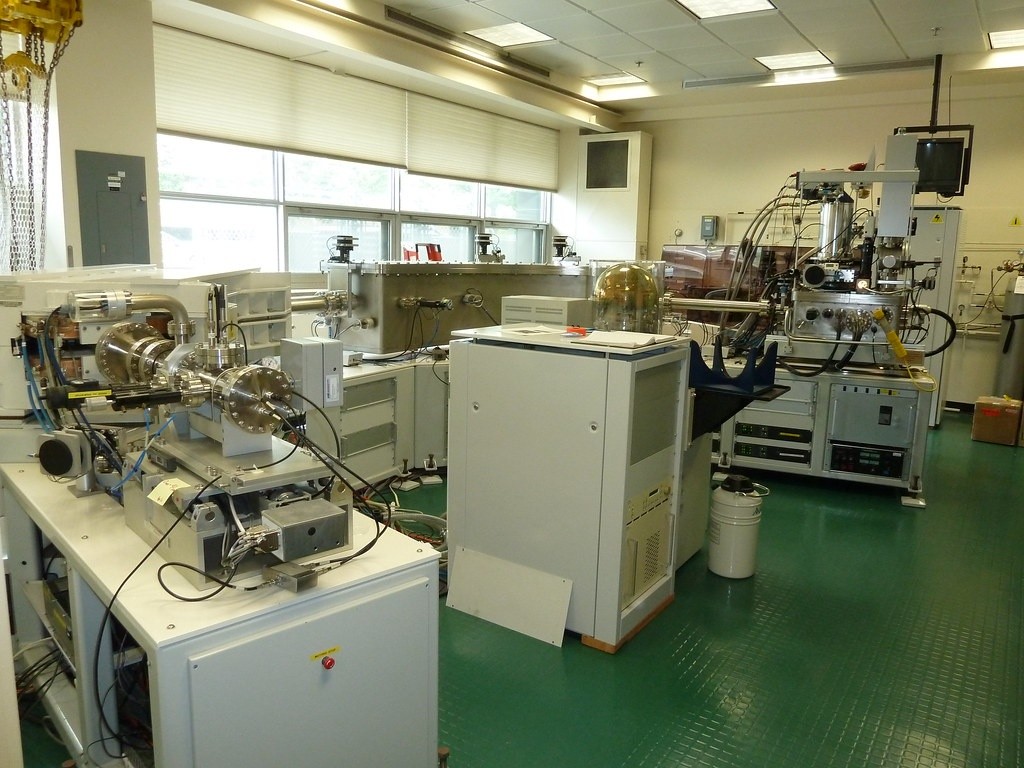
969,393,1023,449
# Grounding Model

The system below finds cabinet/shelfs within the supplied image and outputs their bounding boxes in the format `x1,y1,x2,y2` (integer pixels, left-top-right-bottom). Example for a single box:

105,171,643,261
573,128,650,273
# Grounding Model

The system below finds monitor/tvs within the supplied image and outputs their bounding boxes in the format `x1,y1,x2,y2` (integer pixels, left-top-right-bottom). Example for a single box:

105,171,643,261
912,136,965,193
